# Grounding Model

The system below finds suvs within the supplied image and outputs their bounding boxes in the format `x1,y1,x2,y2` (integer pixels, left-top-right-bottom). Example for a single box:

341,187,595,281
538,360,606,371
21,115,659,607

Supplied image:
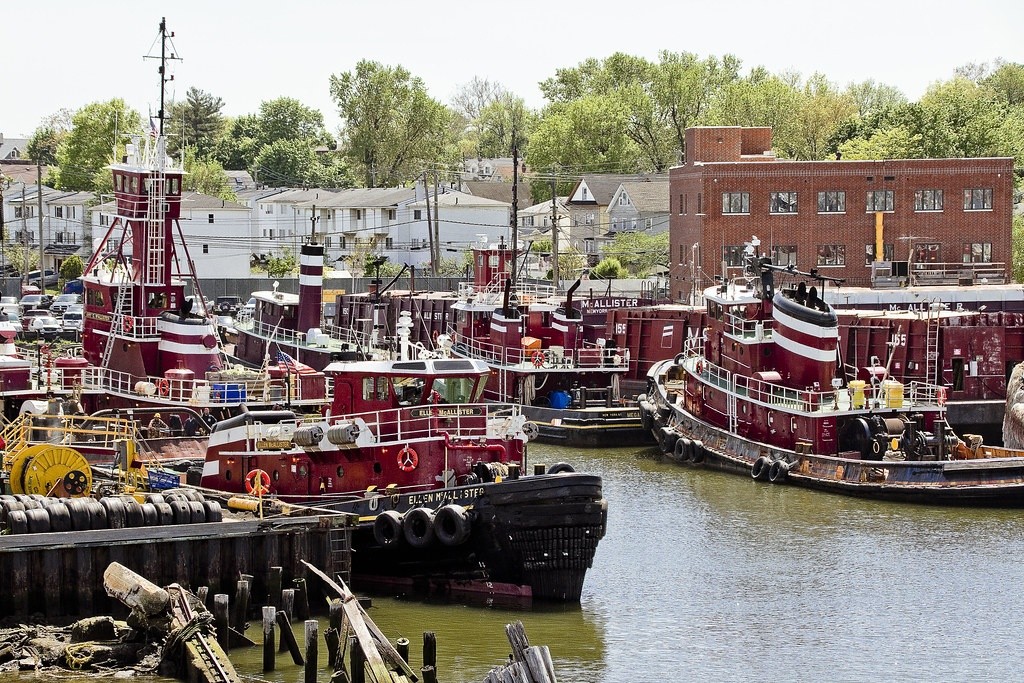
214,296,245,317
47,294,83,314
185,294,214,315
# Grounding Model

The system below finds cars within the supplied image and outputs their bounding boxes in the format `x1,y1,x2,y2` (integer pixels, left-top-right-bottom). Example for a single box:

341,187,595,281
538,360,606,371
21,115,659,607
18,294,52,311
0,295,23,333
19,310,63,333
20,285,40,294
243,298,257,314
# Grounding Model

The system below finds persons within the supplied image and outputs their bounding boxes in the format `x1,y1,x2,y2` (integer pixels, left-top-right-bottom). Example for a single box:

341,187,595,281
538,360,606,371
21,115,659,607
148,413,167,438
184,413,198,436
168,413,182,436
198,408,217,436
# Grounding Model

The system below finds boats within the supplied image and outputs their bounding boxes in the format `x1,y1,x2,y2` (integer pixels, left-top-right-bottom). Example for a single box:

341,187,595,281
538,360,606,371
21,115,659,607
404,145,658,448
636,211,1024,507
183,253,608,607
0,16,342,476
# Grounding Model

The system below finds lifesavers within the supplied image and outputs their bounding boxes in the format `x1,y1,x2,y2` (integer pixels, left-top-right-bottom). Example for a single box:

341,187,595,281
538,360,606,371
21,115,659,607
159,378,170,396
122,316,135,332
432,330,439,343
546,462,576,475
433,504,472,548
750,455,772,483
243,469,270,497
373,509,406,549
531,351,545,367
396,447,418,473
403,507,438,550
0,487,223,535
658,427,704,464
768,460,789,484
695,360,704,377
637,393,655,432
602,499,608,537
936,388,947,406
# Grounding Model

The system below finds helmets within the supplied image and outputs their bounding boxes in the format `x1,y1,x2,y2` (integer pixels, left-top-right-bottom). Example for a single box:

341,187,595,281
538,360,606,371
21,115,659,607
154,412,161,418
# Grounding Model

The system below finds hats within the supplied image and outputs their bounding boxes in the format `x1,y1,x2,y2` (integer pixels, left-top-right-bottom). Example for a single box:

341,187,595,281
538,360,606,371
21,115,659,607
205,408,209,412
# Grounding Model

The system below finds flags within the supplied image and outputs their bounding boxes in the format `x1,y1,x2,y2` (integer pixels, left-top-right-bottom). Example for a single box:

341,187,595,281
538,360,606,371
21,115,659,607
277,351,316,375
150,118,159,138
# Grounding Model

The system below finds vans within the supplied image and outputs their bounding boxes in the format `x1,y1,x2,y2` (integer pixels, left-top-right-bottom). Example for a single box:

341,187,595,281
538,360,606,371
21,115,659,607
23,269,59,288
63,303,83,330
